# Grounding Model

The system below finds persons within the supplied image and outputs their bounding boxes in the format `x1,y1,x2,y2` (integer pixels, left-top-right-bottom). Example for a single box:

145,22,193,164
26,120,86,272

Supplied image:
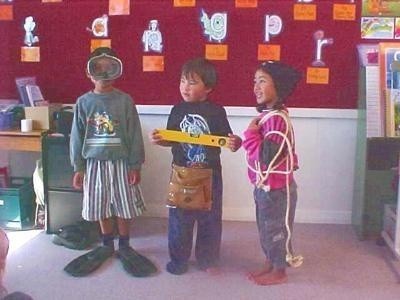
243,59,302,285
147,56,242,277
71,45,144,262
0,227,35,299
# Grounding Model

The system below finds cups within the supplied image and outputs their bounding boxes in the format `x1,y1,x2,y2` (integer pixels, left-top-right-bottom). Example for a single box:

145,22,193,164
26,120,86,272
21,119,33,133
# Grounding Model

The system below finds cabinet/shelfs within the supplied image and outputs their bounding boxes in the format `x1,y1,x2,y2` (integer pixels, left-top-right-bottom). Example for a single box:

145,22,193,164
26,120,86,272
351,65,400,261
0,130,91,234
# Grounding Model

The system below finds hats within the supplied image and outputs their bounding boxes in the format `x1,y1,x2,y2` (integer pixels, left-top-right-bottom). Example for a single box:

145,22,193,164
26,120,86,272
257,59,304,100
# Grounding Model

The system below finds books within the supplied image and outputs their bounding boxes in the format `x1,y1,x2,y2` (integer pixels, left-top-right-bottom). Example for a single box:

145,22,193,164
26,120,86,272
357,41,400,139
26,85,45,107
15,77,38,107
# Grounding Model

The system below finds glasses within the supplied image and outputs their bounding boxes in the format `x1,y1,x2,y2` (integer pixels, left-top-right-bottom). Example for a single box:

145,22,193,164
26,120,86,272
83,52,124,82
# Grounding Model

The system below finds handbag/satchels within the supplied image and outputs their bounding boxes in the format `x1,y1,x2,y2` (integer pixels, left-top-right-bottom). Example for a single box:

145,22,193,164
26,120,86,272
164,160,215,212
